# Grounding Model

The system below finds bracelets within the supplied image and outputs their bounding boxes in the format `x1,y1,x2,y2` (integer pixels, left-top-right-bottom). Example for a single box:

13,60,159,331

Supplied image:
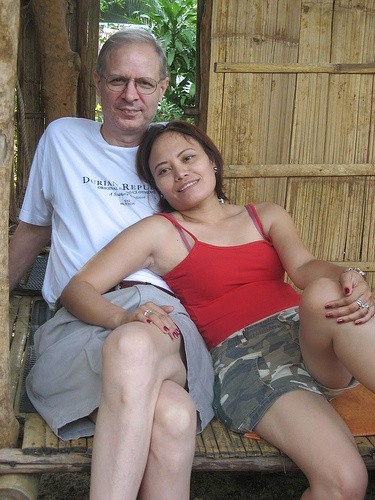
342,266,368,281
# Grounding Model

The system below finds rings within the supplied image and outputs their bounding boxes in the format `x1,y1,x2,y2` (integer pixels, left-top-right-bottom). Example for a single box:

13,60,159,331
361,304,369,309
356,299,363,307
143,309,152,315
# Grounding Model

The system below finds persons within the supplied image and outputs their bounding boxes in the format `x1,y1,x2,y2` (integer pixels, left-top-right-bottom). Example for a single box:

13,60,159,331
60,119,375,500
9,25,217,499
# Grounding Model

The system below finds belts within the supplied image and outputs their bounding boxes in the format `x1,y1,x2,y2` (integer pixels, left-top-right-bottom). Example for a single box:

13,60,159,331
102,280,178,297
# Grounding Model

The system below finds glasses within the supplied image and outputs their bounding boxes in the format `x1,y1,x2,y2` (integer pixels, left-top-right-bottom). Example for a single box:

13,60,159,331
95,69,165,95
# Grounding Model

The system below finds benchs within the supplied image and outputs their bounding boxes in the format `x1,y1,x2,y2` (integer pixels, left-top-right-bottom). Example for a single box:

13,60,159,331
6,287,374,476
